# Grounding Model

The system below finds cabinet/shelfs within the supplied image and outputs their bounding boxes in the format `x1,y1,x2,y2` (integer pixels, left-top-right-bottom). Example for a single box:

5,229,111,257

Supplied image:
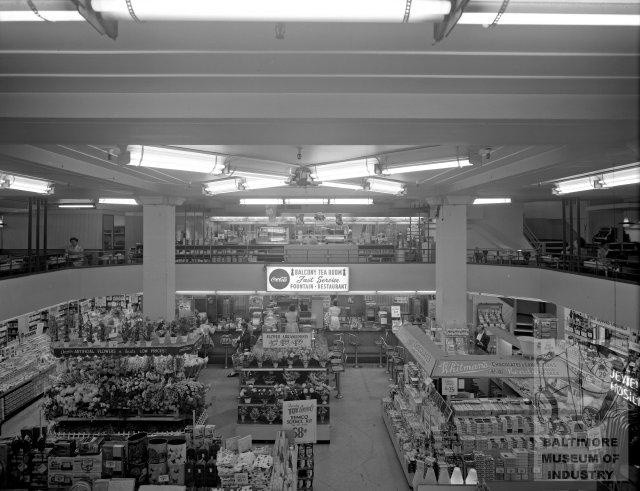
372,290,640,490
0,292,359,491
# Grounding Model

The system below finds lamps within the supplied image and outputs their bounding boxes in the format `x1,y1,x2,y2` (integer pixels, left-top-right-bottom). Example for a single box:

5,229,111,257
116,139,475,196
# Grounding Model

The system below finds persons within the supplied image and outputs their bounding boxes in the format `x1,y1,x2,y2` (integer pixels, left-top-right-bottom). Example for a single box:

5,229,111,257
530,339,632,451
597,240,614,260
243,318,254,342
302,231,319,245
64,236,86,267
473,323,492,353
225,320,252,378
199,316,217,349
284,305,301,333
344,227,353,243
561,237,588,260
327,299,341,333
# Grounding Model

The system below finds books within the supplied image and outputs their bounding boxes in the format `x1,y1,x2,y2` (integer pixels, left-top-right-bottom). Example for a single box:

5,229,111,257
469,339,480,346
